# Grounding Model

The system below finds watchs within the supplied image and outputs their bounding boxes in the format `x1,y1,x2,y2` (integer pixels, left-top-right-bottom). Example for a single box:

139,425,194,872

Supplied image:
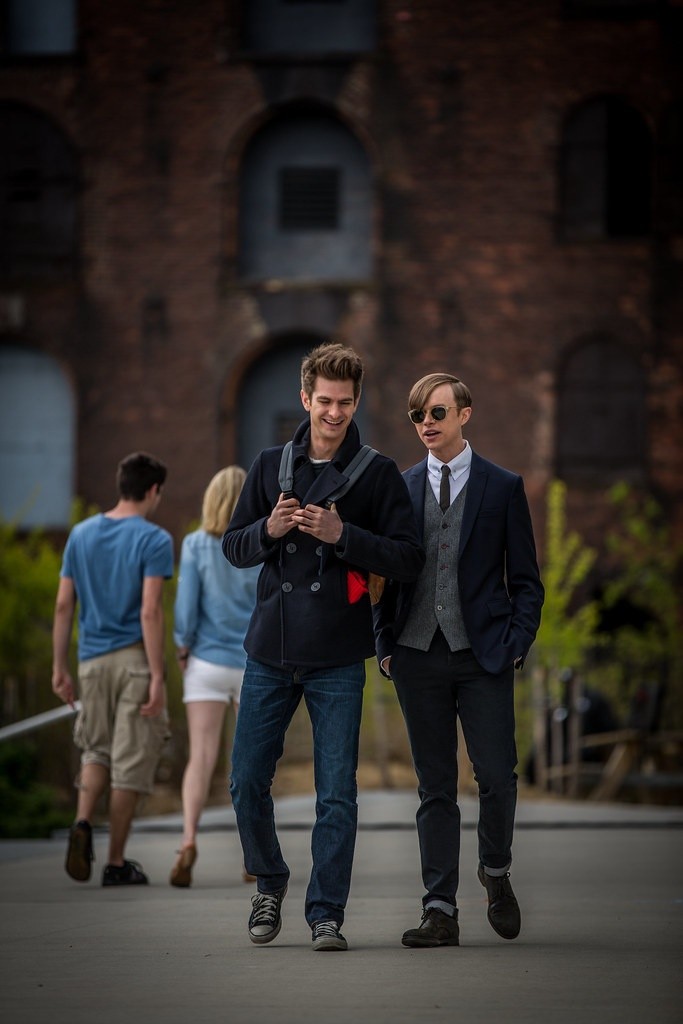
177,653,187,659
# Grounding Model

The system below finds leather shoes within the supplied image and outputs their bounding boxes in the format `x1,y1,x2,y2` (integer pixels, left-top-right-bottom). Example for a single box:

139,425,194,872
477,863,521,939
401,908,460,946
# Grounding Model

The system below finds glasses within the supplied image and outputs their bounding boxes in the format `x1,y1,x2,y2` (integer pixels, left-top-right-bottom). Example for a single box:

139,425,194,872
407,404,465,425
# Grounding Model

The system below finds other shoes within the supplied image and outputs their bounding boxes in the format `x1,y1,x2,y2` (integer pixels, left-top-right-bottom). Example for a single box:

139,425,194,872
170,847,196,887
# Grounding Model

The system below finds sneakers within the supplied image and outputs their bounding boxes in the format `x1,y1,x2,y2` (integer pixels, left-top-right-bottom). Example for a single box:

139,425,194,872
67,822,94,882
311,920,348,952
248,884,287,942
102,862,147,887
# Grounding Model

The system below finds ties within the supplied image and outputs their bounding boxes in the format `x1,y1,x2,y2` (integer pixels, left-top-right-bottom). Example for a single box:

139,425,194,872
440,464,451,512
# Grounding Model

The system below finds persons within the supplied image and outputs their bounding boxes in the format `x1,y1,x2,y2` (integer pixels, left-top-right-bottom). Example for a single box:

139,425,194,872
49,452,174,886
169,466,266,889
367,373,544,947
220,342,422,950
